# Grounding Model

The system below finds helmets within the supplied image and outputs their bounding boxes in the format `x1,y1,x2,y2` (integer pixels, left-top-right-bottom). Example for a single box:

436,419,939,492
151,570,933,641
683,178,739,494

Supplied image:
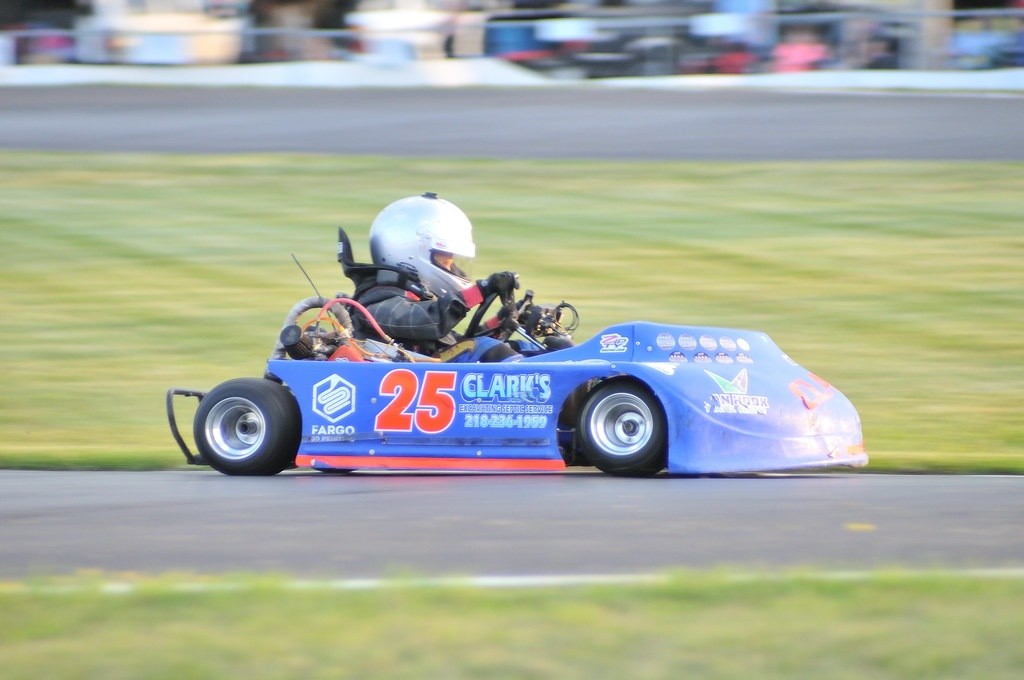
370,192,476,299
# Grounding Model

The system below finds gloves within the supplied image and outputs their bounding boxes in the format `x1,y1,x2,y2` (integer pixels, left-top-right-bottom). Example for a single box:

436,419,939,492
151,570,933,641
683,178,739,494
497,299,542,330
475,271,519,306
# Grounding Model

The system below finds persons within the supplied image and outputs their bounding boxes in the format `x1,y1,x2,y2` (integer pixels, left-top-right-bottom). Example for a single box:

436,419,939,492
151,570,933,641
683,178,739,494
337,192,527,363
